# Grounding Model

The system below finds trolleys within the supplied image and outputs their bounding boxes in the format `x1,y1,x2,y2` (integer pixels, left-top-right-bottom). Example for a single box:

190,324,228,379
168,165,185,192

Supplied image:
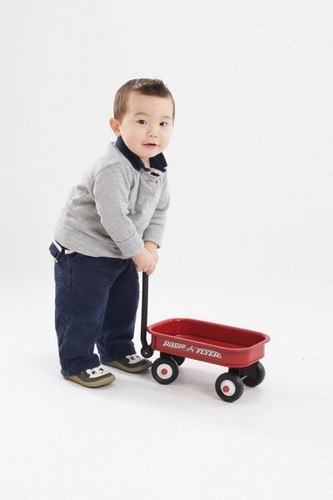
141,271,270,401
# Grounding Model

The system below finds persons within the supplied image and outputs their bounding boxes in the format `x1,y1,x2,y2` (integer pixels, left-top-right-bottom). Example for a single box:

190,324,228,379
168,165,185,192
52,77,175,389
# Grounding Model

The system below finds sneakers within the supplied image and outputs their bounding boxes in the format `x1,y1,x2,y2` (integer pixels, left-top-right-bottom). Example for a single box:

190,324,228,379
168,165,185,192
64,366,114,387
105,354,153,372
